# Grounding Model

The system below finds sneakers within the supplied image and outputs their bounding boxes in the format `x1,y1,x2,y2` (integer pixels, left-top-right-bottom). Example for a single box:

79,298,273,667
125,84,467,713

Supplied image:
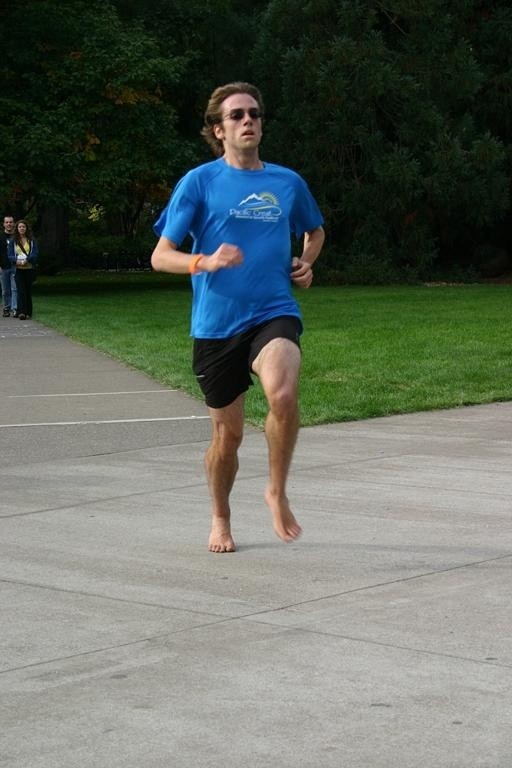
2,308,31,319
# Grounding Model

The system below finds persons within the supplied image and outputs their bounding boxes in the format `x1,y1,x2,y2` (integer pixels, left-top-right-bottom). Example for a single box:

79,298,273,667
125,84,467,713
6,219,39,320
149,81,328,553
0,216,16,318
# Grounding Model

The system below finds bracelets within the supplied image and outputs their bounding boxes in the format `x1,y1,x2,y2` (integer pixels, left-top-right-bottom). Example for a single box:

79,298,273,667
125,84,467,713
189,253,205,274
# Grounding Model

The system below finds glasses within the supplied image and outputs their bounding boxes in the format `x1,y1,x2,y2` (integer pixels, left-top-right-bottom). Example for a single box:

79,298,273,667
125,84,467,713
223,108,262,120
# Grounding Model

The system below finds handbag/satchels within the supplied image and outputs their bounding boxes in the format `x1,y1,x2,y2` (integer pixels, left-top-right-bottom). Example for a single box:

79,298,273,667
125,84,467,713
32,263,39,282
